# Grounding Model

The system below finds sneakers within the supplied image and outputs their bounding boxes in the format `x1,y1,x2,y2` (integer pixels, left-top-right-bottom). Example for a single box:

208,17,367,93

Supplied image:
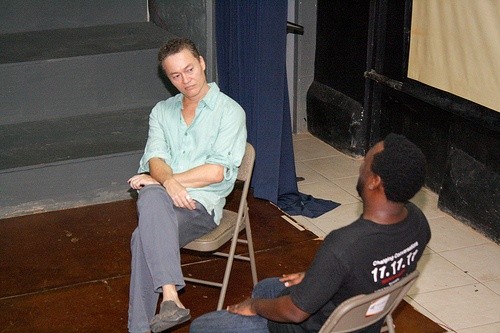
150,301,192,333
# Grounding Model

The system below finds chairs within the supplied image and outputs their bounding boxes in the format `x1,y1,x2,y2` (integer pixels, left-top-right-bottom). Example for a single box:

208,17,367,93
178,142,258,311
316,269,419,333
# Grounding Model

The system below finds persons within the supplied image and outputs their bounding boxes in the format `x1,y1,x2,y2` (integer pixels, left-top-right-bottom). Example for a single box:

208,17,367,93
189,132,433,333
127,36,248,333
